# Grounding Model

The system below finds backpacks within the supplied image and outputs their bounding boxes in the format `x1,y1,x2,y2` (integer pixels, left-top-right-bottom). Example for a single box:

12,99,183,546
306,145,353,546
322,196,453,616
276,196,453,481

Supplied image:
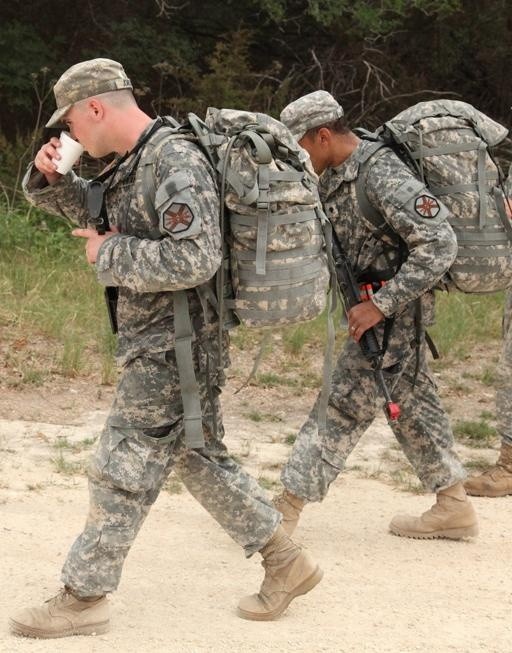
131,102,331,333
353,94,510,296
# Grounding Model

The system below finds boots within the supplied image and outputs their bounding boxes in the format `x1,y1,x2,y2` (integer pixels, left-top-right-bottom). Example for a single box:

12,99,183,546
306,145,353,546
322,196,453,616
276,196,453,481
390,480,480,538
461,435,512,497
268,483,304,537
232,523,323,620
8,584,111,639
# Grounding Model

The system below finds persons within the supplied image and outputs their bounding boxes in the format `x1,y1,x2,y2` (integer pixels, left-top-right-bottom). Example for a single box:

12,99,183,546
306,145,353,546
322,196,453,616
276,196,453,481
463,149,512,498
8,57,328,639
267,89,482,540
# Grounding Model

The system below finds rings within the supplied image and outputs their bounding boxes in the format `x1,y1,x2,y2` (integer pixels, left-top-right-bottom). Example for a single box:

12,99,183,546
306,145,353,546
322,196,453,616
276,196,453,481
350,326,356,332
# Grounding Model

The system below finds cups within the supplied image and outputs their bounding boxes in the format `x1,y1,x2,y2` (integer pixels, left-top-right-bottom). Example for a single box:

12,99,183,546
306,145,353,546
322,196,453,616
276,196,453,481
44,131,81,177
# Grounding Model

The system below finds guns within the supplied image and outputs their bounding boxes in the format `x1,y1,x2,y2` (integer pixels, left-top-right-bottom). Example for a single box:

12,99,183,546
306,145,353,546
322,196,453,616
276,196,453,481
88,181,119,335
332,230,401,420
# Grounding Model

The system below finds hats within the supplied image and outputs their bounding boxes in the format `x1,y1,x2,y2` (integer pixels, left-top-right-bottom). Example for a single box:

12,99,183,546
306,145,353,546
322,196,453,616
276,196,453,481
279,89,346,146
44,55,134,131
